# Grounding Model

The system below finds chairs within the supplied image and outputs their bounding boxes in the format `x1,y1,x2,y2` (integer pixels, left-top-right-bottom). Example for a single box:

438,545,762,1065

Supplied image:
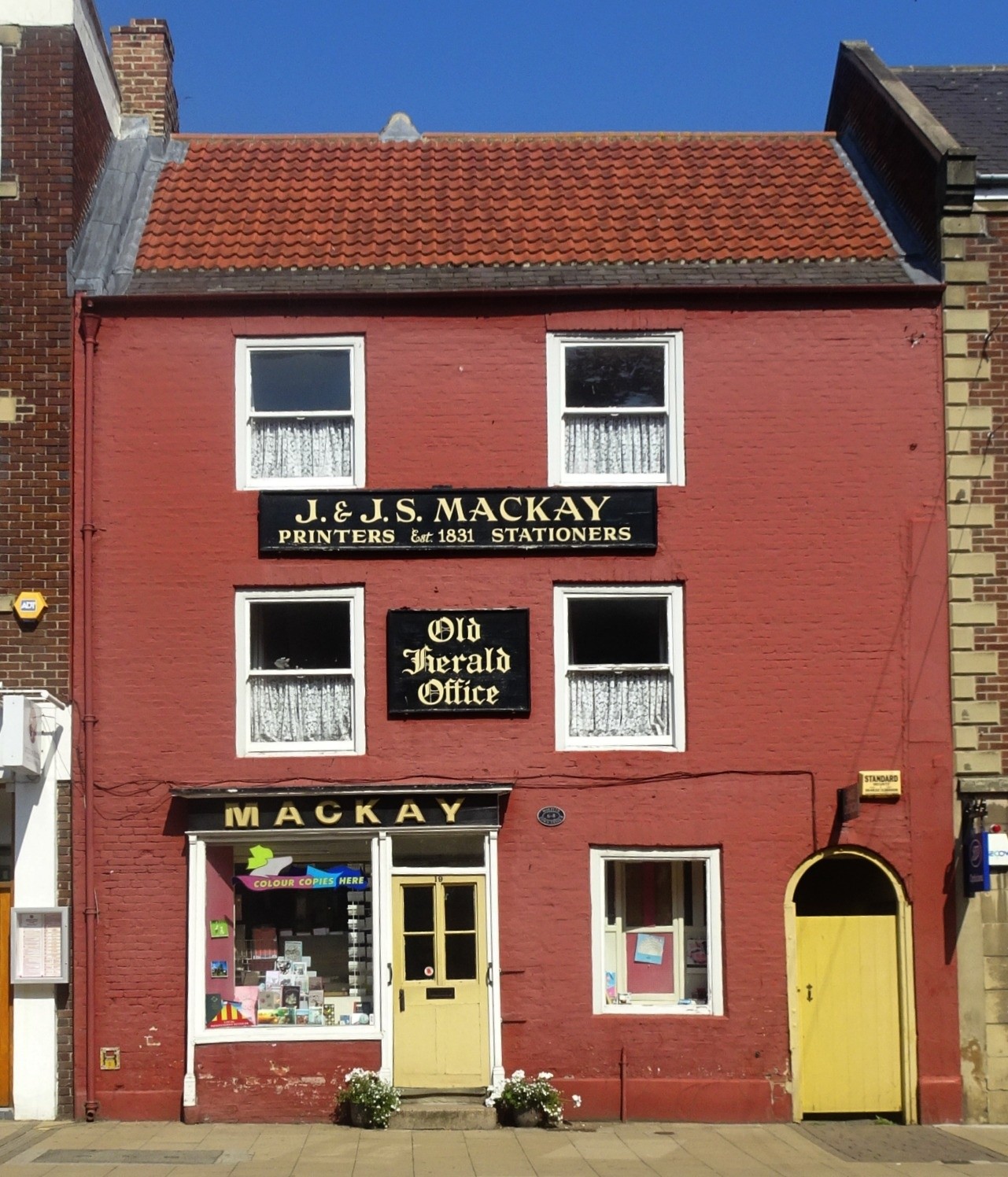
241,971,259,986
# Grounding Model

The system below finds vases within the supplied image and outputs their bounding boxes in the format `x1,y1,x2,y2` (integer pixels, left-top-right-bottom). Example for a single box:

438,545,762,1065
350,1100,376,1127
513,1101,544,1127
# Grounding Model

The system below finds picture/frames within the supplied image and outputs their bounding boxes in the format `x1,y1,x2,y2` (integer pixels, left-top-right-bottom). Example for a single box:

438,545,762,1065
211,961,228,978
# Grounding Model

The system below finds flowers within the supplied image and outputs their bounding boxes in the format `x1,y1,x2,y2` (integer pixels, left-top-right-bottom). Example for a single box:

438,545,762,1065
485,1068,582,1122
332,1066,402,1131
